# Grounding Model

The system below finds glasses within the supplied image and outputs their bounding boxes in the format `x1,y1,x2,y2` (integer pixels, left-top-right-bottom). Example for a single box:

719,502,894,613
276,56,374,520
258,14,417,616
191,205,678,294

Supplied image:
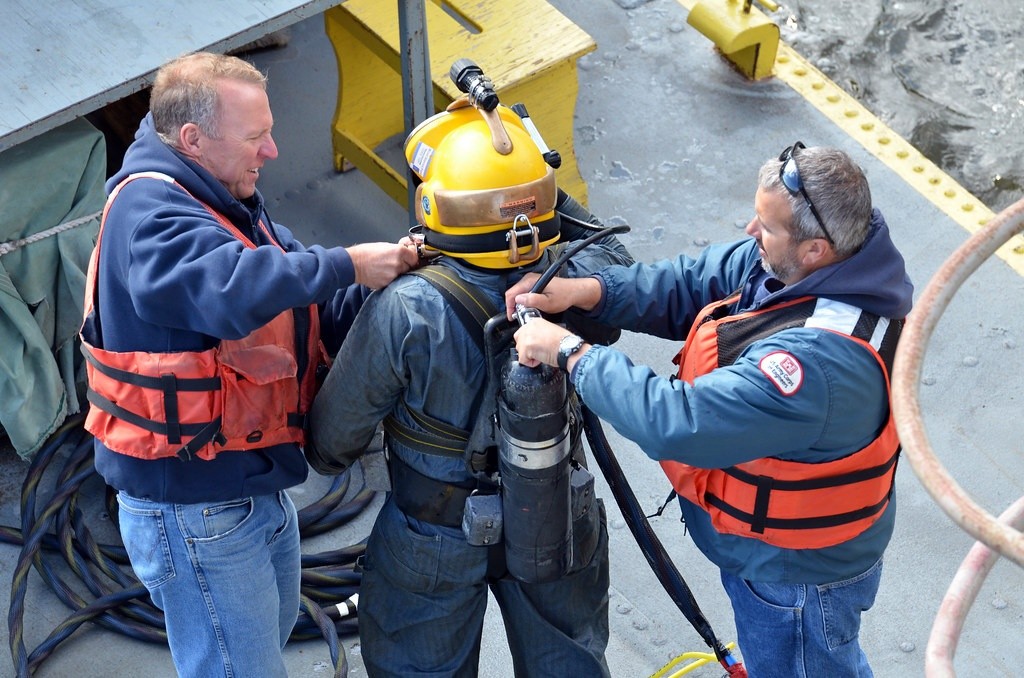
779,140,835,245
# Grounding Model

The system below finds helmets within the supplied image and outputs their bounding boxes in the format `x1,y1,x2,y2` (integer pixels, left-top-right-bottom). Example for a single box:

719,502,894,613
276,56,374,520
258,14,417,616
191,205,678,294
402,94,561,269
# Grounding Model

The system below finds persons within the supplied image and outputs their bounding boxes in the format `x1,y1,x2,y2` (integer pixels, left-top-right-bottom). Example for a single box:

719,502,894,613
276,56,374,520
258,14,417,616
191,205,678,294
303,58,636,678
78,50,437,678
505,141,916,678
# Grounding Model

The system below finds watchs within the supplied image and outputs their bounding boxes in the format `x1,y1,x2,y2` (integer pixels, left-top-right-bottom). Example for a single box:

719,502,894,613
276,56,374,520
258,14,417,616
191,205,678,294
556,334,585,376
